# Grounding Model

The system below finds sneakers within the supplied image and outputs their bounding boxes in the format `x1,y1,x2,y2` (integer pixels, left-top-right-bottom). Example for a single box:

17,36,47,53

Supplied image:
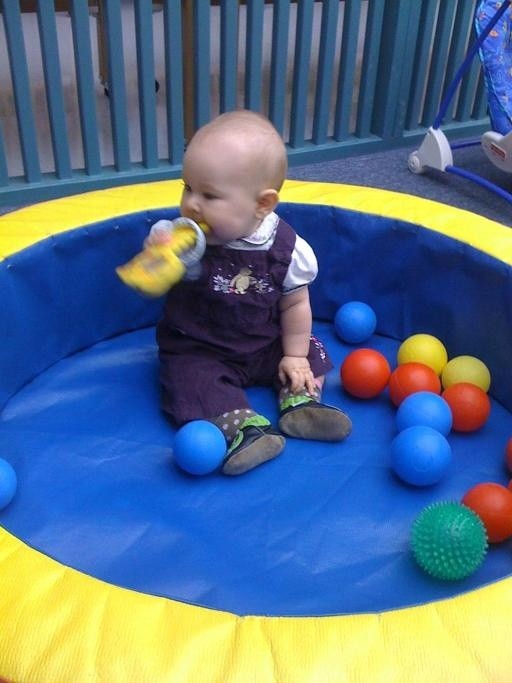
217,413,289,476
277,395,353,444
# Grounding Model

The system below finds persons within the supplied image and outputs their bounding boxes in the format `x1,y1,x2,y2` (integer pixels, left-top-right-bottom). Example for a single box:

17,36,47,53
148,110,354,477
